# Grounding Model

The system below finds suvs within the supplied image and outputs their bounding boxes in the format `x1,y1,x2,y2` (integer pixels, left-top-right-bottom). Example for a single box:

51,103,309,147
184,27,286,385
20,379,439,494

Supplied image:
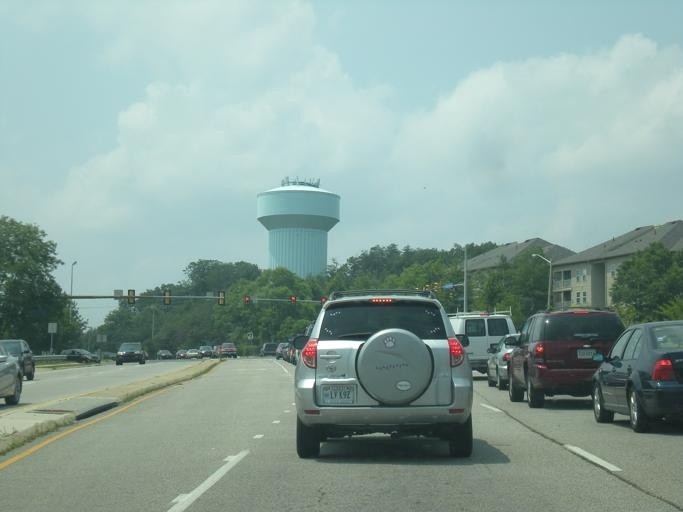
59,348,100,364
291,286,471,460
114,342,145,366
0,338,35,381
501,310,627,408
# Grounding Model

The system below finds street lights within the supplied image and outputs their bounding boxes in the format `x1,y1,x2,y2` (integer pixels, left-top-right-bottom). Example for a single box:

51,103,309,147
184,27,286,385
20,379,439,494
531,253,552,311
68,259,77,323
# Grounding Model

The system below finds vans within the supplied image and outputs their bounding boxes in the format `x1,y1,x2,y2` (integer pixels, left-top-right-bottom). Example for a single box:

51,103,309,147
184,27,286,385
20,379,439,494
446,311,516,374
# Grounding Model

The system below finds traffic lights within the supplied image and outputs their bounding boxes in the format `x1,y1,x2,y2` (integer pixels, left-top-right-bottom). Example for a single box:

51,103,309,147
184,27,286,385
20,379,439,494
245,294,250,307
289,295,296,307
319,296,326,309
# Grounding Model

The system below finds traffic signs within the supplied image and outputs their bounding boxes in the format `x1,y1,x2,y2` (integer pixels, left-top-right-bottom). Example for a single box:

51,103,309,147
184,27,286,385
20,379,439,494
441,283,453,289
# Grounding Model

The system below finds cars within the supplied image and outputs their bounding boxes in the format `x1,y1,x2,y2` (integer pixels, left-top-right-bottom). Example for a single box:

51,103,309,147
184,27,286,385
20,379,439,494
156,349,173,359
144,351,148,359
111,353,116,361
591,320,682,431
259,322,314,367
486,332,521,391
0,341,24,407
174,341,239,359
